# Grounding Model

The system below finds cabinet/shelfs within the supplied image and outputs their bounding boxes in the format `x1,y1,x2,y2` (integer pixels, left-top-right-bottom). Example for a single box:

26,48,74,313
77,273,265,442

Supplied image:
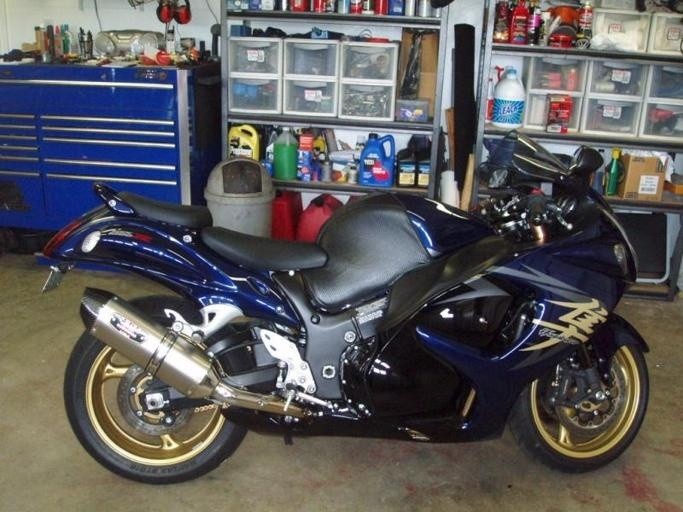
473,42,683,214
221,9,448,199
0,56,221,235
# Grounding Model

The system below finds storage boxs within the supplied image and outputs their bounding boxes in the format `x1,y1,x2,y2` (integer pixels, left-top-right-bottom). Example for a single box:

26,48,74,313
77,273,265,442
227,37,399,122
644,61,683,102
396,97,431,123
522,9,683,144
617,154,666,202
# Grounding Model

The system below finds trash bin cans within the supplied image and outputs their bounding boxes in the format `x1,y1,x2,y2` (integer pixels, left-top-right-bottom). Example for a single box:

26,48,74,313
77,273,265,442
205,158,275,238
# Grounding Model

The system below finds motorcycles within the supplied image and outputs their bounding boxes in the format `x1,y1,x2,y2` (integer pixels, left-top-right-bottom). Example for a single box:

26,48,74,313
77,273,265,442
33,127,649,485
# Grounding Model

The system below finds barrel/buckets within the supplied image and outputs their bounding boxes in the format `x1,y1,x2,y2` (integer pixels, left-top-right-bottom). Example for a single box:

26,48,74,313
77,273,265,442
295,191,342,241
271,189,302,242
490,67,528,128
228,125,259,160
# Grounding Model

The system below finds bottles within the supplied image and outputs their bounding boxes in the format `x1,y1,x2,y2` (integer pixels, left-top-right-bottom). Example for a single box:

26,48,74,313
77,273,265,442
593,147,606,196
567,69,577,91
492,69,525,128
606,149,622,197
539,11,549,51
347,165,358,185
511,0,526,44
485,78,492,124
528,9,538,44
